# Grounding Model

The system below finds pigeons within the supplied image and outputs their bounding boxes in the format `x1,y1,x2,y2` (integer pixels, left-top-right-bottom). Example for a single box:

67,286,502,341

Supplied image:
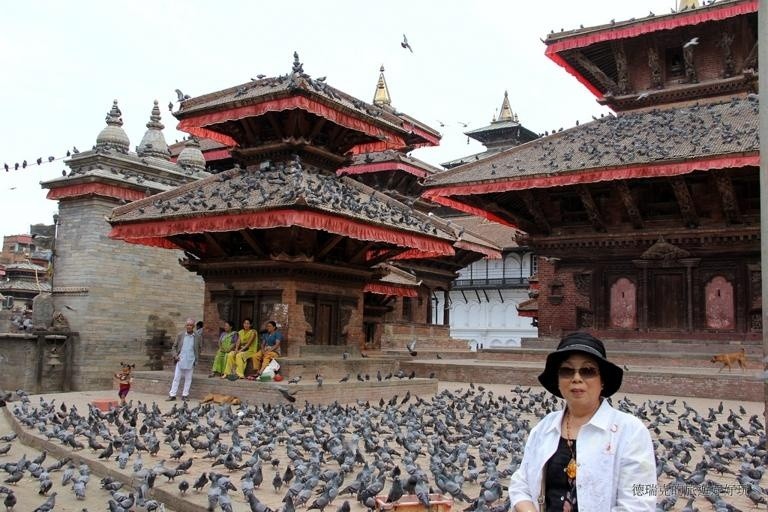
681,36,700,49
401,33,413,54
0,336,768,512
4,64,760,263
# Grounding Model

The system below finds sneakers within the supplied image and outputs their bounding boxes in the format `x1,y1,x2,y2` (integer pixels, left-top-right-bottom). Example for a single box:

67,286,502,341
208,372,227,379
119,402,127,408
182,396,189,401
166,396,176,401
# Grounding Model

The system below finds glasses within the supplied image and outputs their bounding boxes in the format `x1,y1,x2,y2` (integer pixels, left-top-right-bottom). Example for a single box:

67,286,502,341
558,365,597,379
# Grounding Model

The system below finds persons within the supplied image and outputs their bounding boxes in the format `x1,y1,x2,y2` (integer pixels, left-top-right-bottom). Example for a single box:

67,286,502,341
195,321,204,350
508,331,656,512
208,320,238,380
114,362,136,408
220,318,259,379
165,318,200,404
248,321,282,380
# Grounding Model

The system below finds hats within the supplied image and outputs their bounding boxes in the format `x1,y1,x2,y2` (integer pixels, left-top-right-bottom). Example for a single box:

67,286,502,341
538,333,622,398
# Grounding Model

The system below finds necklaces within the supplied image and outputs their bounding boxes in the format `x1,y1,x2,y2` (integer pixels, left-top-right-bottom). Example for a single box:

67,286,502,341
566,412,578,480
